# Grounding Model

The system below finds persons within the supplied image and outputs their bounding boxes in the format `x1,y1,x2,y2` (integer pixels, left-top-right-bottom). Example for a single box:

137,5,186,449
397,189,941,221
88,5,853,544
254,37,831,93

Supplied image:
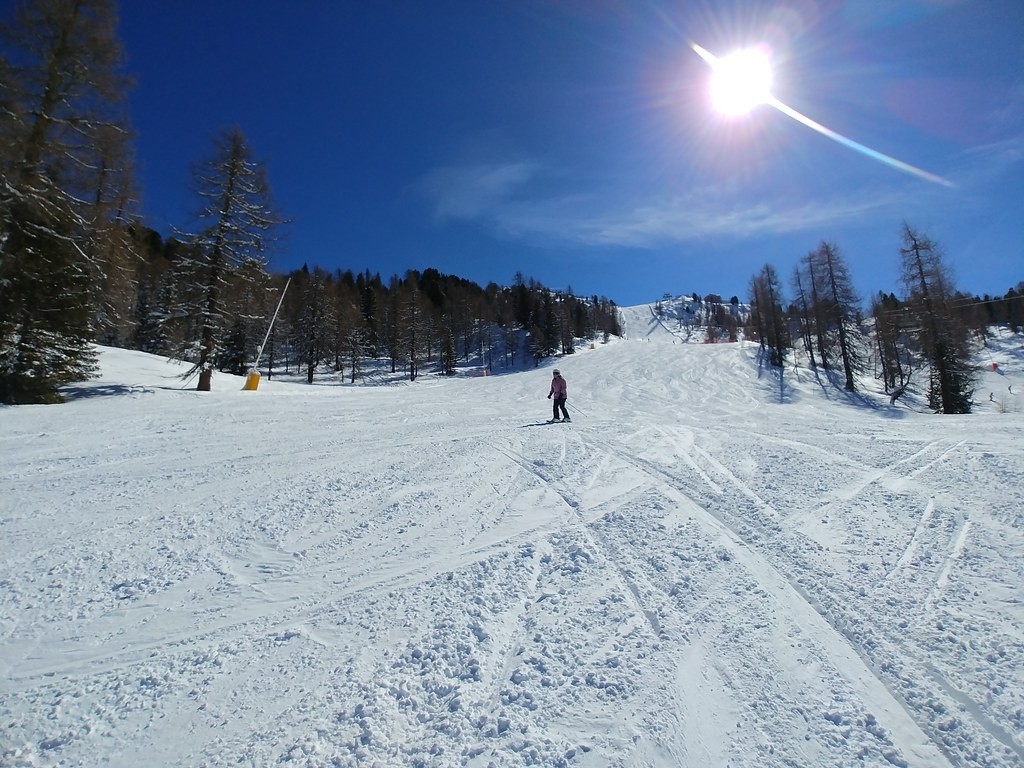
1008,385,1011,393
547,369,572,423
989,392,994,400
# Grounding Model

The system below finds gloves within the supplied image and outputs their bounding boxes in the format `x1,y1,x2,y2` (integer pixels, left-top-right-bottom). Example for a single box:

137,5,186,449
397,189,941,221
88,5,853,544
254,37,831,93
558,393,563,400
548,393,552,399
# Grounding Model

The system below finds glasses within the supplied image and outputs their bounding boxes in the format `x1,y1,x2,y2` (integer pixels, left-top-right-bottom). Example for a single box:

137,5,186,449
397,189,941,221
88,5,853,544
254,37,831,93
553,372,559,375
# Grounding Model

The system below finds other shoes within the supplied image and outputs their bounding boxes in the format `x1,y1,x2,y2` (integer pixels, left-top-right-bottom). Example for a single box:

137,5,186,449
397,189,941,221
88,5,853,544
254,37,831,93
551,418,560,422
563,418,570,422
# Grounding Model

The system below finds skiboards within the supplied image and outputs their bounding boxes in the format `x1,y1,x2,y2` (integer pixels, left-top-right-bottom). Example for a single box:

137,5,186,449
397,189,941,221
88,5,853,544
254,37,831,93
546,420,571,422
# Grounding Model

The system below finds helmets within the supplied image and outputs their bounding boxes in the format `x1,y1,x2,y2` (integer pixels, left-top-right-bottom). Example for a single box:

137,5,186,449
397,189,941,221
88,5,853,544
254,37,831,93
552,368,560,376
